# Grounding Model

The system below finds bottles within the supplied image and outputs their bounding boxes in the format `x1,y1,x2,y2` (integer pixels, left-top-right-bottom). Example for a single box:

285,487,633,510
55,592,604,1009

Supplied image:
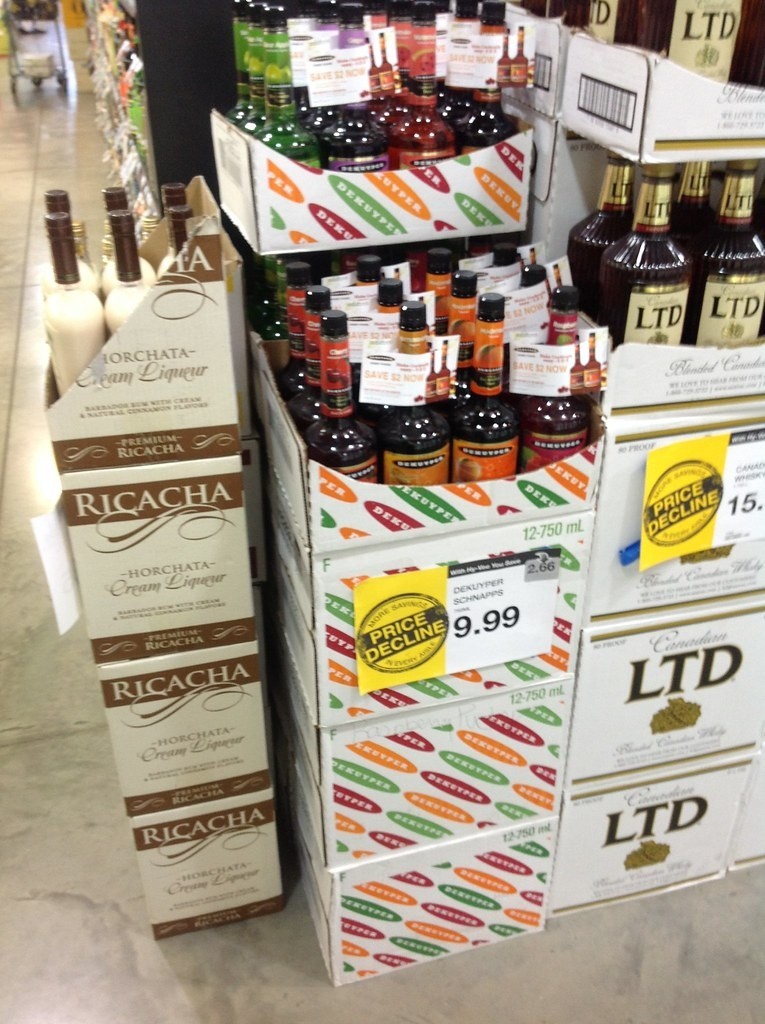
424,338,452,402
363,34,396,101
38,182,204,409
228,220,593,486
570,332,602,395
508,0,764,96
497,24,528,88
565,149,765,360
228,0,518,169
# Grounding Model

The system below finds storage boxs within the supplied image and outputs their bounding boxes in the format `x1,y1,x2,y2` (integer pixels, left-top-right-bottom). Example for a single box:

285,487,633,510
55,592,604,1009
39,173,285,941
209,0,765,986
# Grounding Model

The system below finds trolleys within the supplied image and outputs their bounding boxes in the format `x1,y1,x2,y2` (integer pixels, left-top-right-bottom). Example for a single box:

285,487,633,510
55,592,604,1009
0,0,70,95
